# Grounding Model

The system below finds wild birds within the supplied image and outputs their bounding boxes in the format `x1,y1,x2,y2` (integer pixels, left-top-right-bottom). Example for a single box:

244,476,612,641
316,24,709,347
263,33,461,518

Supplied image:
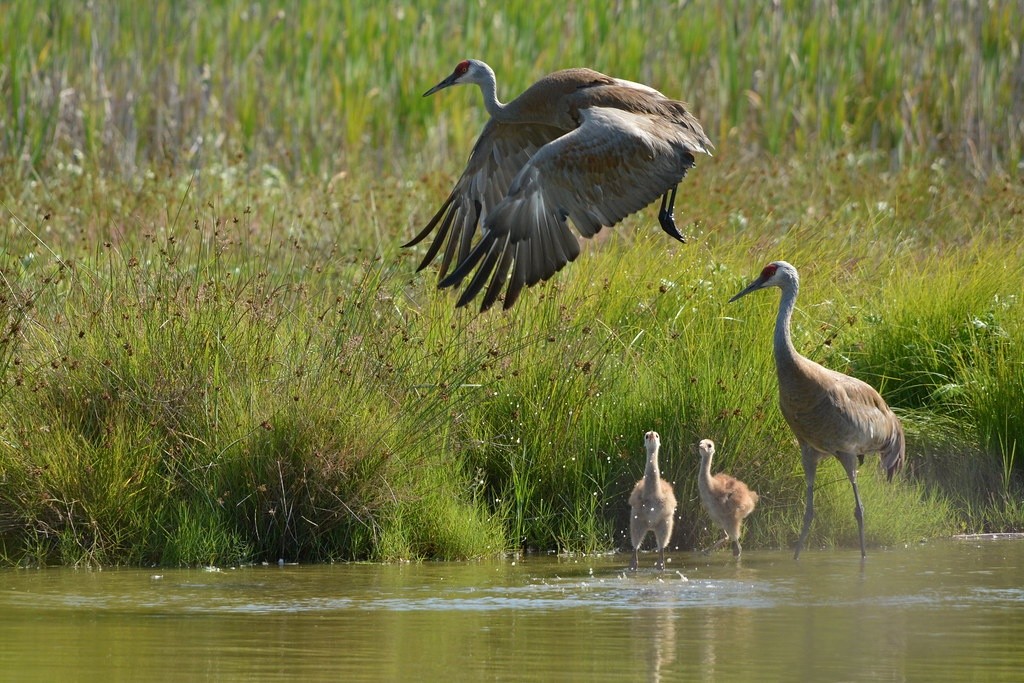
628,431,677,572
698,440,759,557
395,56,714,314
729,261,906,561
